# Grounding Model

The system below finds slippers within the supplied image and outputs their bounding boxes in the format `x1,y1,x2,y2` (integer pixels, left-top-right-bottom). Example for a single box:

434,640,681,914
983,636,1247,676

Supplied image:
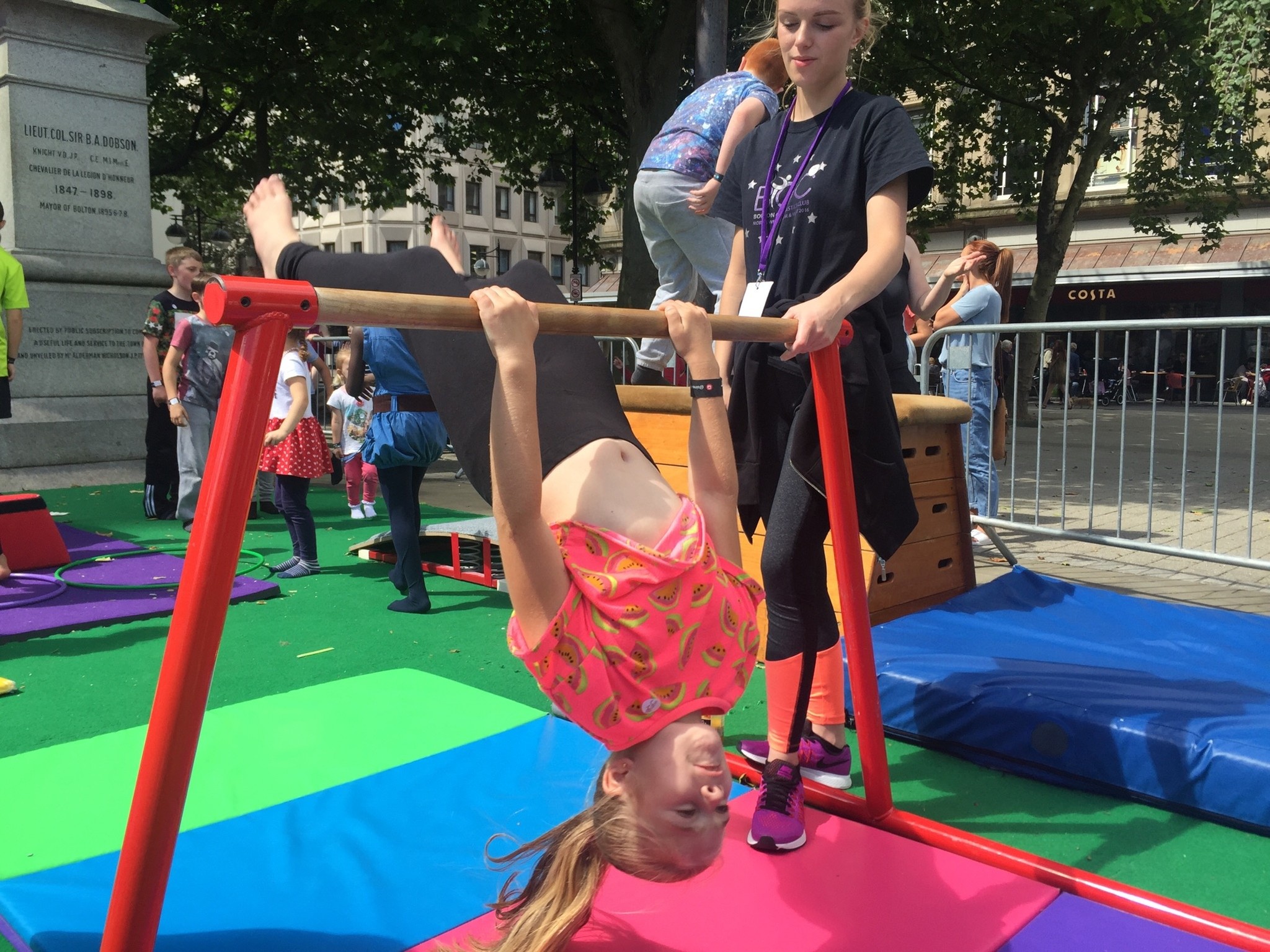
971,529,994,545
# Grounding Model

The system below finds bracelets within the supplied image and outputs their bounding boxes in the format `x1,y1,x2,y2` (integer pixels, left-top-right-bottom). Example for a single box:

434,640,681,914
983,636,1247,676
710,171,724,182
687,380,721,400
6,355,15,364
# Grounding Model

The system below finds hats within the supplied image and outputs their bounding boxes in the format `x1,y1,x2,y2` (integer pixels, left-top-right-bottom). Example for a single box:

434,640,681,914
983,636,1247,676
1071,343,1077,349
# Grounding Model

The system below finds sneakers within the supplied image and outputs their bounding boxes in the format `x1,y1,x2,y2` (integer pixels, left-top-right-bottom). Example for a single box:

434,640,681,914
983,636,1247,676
736,718,852,790
747,760,807,851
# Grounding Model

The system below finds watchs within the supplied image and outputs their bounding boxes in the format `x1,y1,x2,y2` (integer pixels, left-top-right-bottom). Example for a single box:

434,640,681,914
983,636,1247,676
150,380,163,387
167,397,179,406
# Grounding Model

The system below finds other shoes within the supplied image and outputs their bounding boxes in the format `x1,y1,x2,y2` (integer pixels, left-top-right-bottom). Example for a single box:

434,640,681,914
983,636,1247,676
1061,406,1072,409
1035,402,1046,409
1241,399,1252,405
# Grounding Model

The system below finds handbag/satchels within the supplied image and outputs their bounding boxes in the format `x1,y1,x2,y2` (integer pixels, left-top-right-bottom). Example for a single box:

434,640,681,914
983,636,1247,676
993,397,1007,461
1253,374,1267,398
1037,367,1049,377
1089,380,1105,394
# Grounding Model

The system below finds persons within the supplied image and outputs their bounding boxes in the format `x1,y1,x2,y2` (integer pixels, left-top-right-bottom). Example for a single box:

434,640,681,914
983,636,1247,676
712,0,930,858
0,201,29,580
629,40,789,387
145,235,1261,612
241,172,767,952
934,237,1013,546
